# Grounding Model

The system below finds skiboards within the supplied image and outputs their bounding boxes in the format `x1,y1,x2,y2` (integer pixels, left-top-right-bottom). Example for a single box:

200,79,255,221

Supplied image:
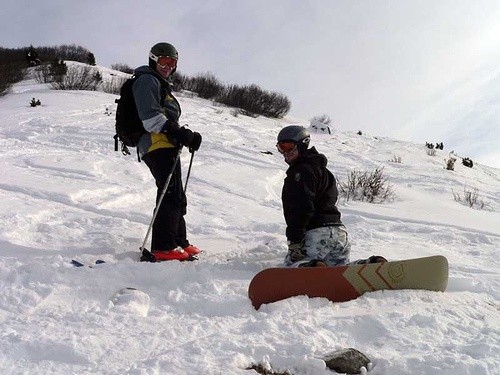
71,249,206,269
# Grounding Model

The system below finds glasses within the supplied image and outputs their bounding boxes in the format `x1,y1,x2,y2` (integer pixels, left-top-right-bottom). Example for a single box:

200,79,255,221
158,57,178,68
276,142,298,154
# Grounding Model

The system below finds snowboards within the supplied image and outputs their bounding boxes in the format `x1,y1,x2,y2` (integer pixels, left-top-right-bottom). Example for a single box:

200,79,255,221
247,254,448,311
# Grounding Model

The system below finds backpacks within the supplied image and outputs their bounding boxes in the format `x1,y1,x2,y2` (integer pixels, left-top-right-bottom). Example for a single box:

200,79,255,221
113,72,167,163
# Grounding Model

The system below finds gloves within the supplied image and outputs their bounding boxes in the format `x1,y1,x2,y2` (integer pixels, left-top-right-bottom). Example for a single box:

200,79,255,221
179,123,201,153
287,239,308,263
161,120,189,148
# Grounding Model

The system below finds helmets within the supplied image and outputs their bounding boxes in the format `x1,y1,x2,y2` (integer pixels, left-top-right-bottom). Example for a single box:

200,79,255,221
149,42,179,75
277,124,310,154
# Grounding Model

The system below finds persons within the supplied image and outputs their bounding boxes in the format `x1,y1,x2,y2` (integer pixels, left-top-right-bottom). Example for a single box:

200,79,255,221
276,125,388,267
130,42,202,261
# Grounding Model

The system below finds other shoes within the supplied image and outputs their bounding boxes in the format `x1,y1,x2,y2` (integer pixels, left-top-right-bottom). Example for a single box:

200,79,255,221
152,246,190,260
184,244,202,256
